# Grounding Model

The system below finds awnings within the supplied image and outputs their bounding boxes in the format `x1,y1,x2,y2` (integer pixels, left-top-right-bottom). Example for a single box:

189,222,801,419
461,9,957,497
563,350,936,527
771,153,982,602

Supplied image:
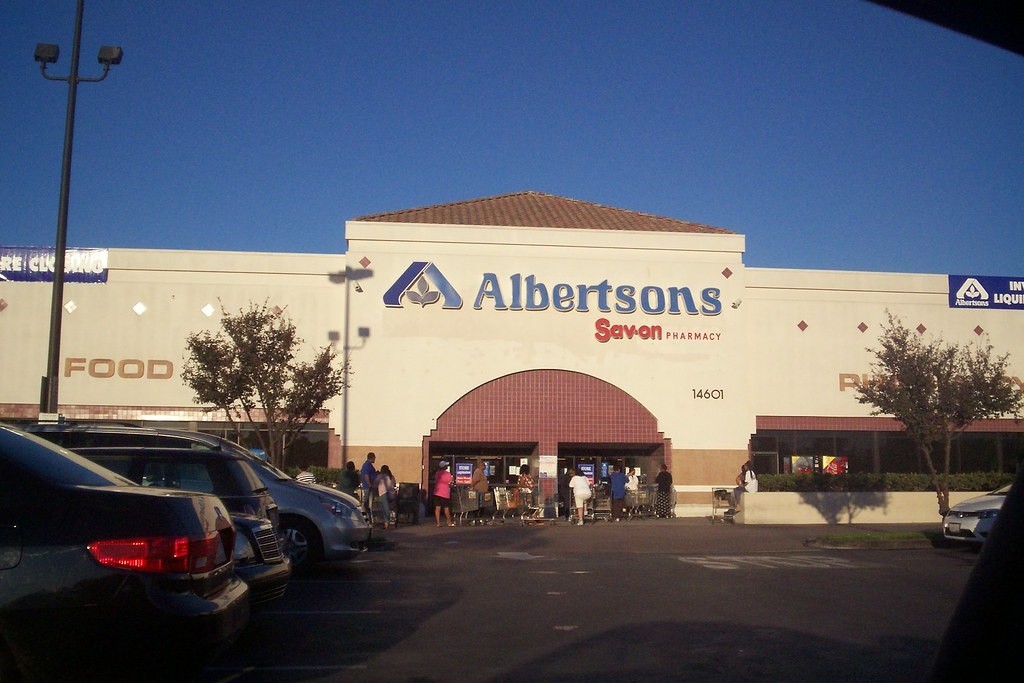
0,403,329,424
756,416,1024,432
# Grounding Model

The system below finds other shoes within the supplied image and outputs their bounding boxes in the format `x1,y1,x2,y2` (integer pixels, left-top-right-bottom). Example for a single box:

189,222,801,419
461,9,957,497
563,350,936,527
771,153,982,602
448,524,454,527
615,517,619,522
584,511,588,516
436,524,442,527
724,510,735,516
657,514,659,519
623,508,626,513
666,515,668,518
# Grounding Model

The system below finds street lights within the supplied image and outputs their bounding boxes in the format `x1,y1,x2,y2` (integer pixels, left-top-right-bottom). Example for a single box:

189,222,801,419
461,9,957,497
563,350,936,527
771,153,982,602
36,1,123,425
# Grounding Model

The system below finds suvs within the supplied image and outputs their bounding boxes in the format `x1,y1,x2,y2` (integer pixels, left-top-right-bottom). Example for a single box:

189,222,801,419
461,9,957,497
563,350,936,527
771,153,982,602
0,418,372,603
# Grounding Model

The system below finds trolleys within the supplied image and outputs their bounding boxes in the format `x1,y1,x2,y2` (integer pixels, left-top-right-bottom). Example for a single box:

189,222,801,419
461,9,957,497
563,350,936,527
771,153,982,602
472,482,677,526
711,487,736,523
443,484,481,527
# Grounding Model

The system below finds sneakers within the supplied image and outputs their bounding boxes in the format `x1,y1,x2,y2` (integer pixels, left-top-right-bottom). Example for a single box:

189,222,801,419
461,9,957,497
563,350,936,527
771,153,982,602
577,521,583,526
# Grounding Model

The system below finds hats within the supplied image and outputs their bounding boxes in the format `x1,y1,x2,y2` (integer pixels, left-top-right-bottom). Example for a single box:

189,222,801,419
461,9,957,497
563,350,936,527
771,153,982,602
439,461,449,468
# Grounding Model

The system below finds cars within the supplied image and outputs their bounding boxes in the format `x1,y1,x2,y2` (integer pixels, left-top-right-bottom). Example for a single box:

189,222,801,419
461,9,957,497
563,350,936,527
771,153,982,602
0,421,251,683
942,479,1015,543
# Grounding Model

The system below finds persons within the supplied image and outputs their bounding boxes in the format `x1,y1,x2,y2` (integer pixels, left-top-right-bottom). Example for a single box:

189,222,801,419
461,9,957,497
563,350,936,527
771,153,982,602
468,461,488,526
145,466,161,486
374,465,396,529
361,452,377,511
517,464,533,507
610,464,639,522
736,460,756,486
432,461,455,527
560,468,592,525
655,464,672,518
339,461,360,493
